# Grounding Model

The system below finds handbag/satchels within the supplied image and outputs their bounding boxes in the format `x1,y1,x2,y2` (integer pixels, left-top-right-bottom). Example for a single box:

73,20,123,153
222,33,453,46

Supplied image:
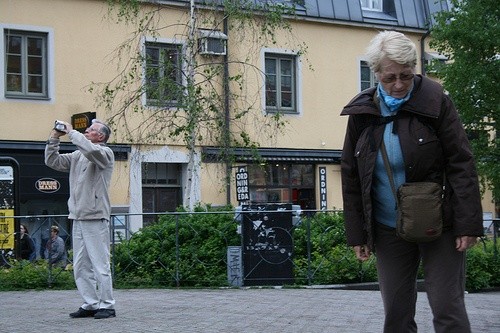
396,182,445,243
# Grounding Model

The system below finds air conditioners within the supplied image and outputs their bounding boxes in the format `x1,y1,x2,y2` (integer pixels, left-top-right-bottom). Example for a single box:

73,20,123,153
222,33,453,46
425,60,446,73
198,37,227,56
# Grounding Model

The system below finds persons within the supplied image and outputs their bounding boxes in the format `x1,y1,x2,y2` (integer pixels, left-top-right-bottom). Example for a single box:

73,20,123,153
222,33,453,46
19,224,36,261
45,119,116,319
44,226,67,267
340,31,485,333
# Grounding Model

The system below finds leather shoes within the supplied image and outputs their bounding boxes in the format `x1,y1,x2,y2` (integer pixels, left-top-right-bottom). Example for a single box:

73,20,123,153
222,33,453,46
94,308,116,319
69,307,99,318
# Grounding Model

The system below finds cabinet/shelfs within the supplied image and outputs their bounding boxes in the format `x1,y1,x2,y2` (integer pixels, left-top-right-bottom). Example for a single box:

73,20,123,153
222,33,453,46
250,163,299,205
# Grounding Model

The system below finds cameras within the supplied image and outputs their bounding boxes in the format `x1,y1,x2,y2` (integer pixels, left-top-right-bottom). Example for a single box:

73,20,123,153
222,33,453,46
56,124,67,130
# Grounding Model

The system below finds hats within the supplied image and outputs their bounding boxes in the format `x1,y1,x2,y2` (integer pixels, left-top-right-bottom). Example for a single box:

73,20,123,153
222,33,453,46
51,225,61,232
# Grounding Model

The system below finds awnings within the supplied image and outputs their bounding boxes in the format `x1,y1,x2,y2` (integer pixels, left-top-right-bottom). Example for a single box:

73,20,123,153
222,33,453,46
0,140,131,154
424,52,448,60
204,147,343,158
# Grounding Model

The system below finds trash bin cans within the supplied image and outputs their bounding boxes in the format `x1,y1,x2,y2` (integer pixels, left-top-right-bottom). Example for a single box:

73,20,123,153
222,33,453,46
242,204,295,286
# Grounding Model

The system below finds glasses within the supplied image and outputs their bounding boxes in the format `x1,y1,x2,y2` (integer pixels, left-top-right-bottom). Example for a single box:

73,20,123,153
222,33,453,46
85,128,104,135
377,70,415,84
